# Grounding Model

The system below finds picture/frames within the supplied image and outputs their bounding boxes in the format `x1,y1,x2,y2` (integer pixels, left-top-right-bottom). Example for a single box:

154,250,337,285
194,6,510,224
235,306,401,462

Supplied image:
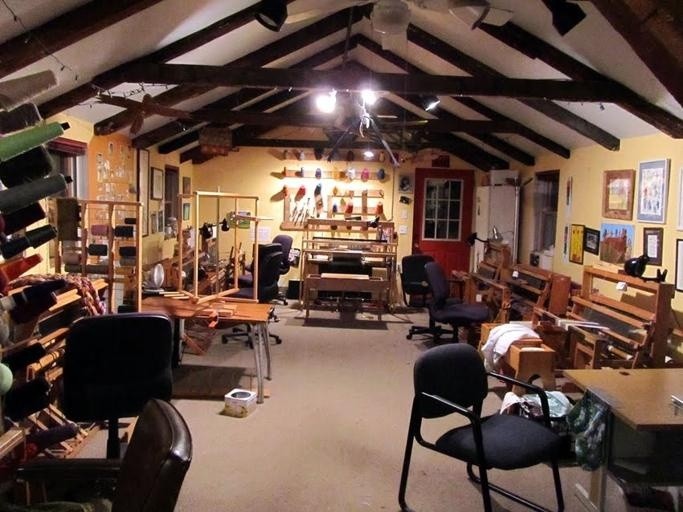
674,166,682,231
151,166,164,202
635,158,670,225
642,227,663,267
183,203,191,221
601,166,636,221
672,238,682,292
568,223,585,265
585,227,599,256
135,145,150,240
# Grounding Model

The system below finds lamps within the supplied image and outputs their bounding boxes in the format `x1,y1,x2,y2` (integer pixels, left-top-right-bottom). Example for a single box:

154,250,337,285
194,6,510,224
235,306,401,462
463,232,491,256
542,1,587,38
368,1,412,37
490,225,514,242
253,0,287,33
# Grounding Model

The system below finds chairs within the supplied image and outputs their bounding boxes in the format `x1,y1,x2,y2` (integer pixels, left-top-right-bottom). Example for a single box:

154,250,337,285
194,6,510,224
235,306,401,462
390,339,570,511
422,259,490,348
219,242,282,348
13,394,193,511
243,233,292,306
395,252,465,345
55,309,178,461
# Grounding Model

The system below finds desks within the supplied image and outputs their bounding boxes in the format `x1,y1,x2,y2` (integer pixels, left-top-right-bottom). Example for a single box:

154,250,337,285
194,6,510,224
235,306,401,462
139,299,276,406
303,273,385,323
558,363,683,512
